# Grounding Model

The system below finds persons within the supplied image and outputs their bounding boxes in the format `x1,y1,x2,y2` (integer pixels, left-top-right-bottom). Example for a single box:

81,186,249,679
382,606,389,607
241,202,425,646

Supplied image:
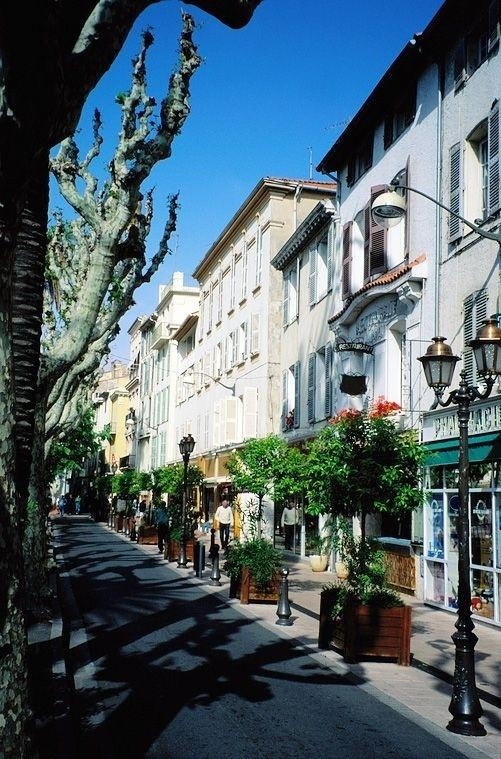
281,500,301,550
215,499,235,549
47,491,83,518
139,500,147,519
154,501,173,554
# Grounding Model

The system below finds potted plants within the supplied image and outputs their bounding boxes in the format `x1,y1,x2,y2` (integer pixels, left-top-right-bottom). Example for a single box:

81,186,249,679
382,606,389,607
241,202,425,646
223,431,302,605
111,468,160,545
299,398,435,661
150,460,203,562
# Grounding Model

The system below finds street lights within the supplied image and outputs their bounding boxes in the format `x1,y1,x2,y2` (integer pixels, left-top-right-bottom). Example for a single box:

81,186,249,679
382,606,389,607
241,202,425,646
110,462,119,530
416,313,501,736
177,434,196,568
